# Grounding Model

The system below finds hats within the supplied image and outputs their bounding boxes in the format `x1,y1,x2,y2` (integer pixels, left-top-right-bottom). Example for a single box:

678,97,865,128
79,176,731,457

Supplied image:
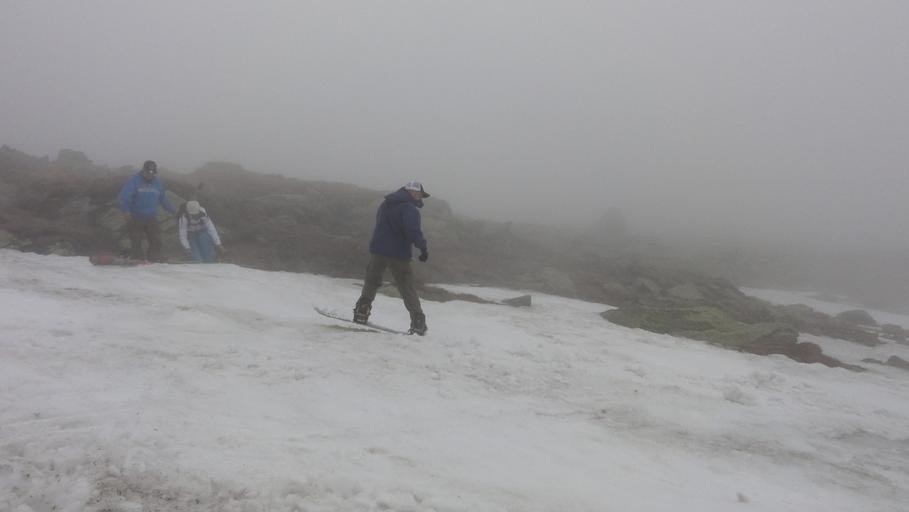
186,201,201,215
404,181,430,198
144,160,156,174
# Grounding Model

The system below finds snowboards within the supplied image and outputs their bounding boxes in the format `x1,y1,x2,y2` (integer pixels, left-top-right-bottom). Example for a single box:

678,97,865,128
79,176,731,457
315,305,411,335
89,254,152,265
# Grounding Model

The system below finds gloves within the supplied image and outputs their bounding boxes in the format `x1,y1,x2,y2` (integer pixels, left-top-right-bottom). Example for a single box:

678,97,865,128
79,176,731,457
420,249,427,261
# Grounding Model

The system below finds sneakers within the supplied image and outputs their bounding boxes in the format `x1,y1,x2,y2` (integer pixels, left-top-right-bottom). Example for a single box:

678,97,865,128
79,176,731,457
355,304,371,315
411,319,425,329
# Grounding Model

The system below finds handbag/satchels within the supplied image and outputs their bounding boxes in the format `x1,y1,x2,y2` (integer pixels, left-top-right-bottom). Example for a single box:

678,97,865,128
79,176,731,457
177,204,190,223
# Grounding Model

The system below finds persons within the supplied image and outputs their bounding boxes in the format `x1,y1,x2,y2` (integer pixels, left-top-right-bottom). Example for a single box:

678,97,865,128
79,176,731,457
117,159,178,263
353,181,431,336
179,200,227,264
